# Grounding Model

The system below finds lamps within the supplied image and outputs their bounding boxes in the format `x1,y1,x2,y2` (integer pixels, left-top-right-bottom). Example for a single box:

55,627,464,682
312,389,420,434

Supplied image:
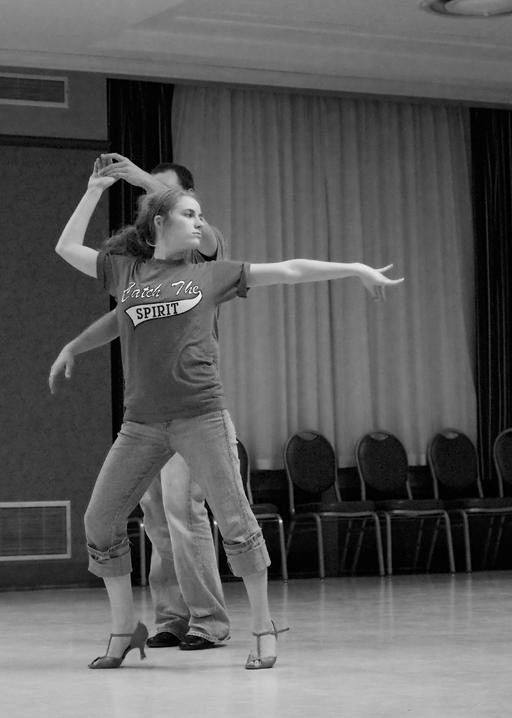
421,0,512,18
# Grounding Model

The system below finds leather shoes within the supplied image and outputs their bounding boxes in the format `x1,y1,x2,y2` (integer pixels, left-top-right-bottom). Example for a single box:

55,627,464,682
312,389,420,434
146,631,180,648
244,619,280,671
179,629,216,651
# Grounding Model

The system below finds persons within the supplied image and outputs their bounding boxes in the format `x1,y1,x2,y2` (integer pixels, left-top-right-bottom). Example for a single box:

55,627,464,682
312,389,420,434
48,153,406,670
47,149,232,651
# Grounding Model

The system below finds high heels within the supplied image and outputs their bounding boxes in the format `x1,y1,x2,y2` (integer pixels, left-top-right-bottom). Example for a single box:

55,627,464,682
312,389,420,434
86,620,150,670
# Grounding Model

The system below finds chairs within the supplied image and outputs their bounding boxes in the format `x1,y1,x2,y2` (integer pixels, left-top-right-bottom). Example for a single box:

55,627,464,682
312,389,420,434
426,429,510,569
281,430,385,580
211,437,289,581
348,430,456,577
128,506,148,582
